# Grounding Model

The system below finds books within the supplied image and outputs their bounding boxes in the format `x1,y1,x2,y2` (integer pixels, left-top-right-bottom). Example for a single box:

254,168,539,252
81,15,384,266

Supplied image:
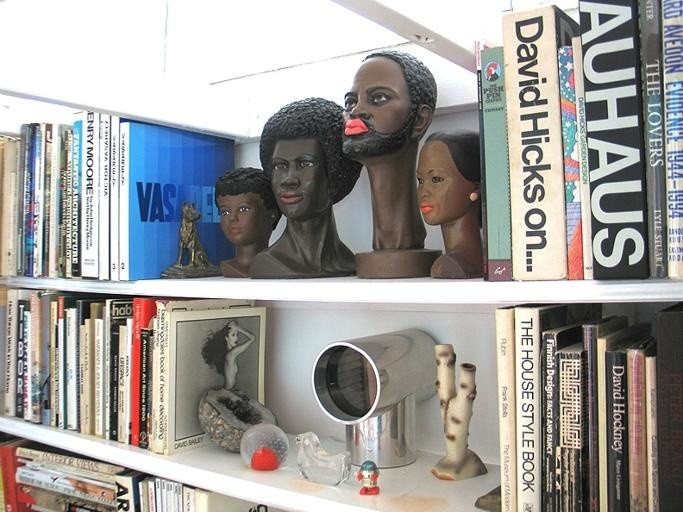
496,301,682,512
0,289,272,512
473,0,683,280
0,109,235,283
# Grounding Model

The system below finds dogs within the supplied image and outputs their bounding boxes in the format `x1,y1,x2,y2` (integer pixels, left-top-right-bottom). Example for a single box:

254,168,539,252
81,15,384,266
175,200,214,273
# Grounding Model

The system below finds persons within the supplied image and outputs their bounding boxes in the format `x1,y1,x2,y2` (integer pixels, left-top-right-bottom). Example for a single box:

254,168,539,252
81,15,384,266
245,96,364,281
211,165,282,278
415,127,485,280
338,49,443,278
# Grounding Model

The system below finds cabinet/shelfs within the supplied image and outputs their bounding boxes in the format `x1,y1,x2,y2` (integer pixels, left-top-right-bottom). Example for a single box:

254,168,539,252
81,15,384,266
0,276,682,510
